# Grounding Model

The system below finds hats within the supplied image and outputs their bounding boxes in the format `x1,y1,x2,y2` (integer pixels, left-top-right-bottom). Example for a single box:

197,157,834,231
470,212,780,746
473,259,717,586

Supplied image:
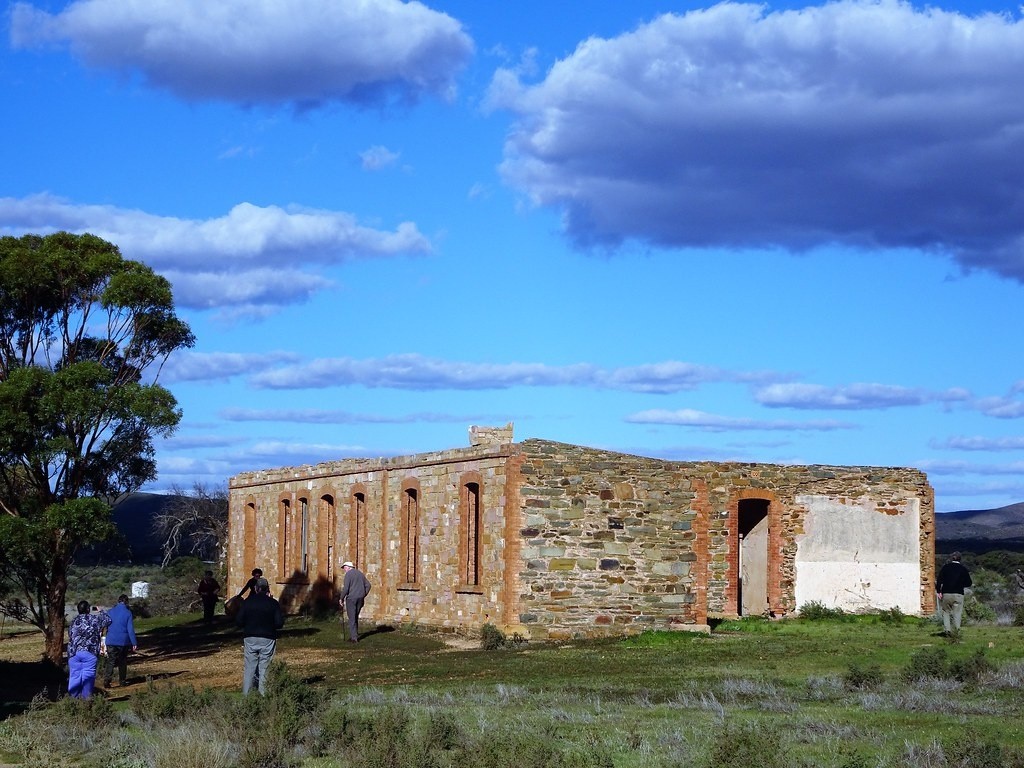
257,578,269,587
341,562,355,569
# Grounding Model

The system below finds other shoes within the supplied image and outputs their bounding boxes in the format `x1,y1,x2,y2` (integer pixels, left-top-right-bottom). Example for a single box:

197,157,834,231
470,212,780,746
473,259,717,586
104,681,111,689
346,638,356,643
120,681,130,687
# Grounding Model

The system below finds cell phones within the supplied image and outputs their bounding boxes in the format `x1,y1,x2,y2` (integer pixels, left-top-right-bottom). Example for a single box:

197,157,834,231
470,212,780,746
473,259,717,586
91,607,97,611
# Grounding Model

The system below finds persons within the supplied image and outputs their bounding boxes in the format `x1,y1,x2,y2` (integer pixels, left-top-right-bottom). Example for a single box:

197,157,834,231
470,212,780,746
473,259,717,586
236,568,285,696
937,551,972,638
198,571,221,625
68,594,138,699
339,562,371,643
